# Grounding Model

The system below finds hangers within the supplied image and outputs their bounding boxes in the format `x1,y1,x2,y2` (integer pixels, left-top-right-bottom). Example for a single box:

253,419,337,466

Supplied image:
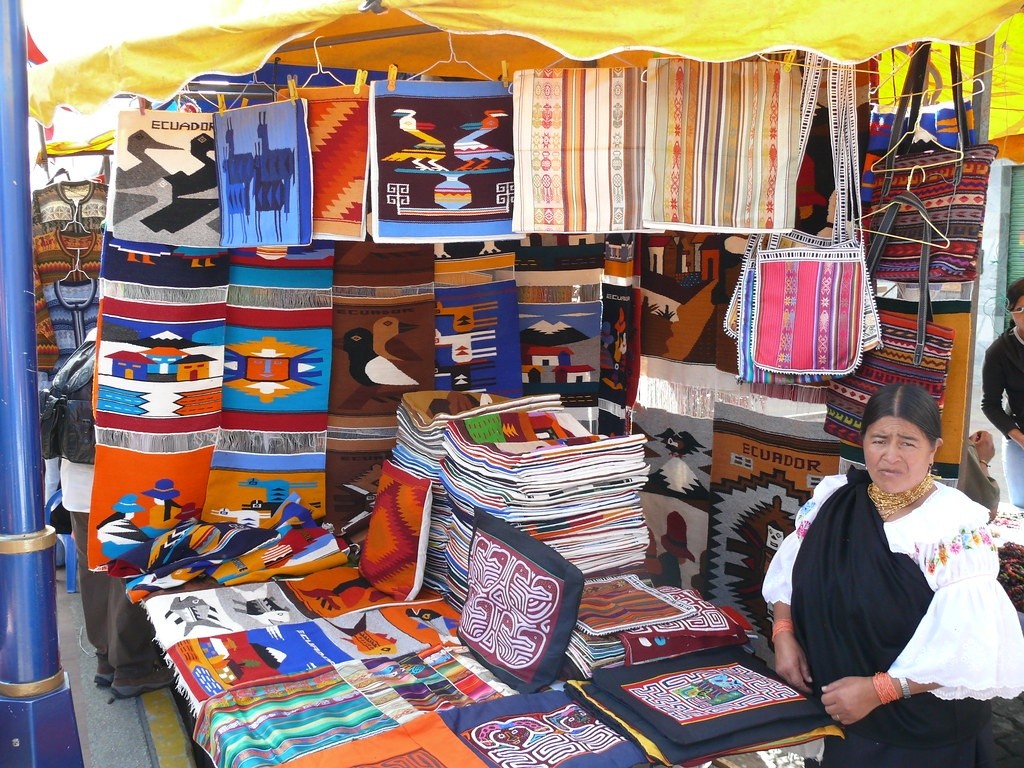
760,39,1003,250
126,27,649,88
59,204,91,301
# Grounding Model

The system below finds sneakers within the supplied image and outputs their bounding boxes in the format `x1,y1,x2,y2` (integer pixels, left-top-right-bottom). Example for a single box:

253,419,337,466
111,662,175,699
94,653,118,686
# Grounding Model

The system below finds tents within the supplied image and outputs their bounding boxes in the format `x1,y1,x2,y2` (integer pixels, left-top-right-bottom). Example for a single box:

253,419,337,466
28,1,1024,141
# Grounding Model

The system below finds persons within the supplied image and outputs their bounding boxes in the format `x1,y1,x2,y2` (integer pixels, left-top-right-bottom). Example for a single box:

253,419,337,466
761,382,1024,768
965,431,1000,522
981,277,1024,509
46,457,75,539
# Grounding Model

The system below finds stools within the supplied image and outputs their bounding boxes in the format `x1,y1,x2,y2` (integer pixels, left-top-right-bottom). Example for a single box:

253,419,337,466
56,534,78,593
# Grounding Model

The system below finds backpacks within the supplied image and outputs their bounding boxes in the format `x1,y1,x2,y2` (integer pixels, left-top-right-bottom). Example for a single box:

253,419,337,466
39,341,96,465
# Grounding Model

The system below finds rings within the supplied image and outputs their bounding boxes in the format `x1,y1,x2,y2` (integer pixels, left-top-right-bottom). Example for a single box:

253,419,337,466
836,714,840,720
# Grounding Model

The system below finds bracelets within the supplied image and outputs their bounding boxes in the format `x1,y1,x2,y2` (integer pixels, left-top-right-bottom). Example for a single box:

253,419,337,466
772,619,794,641
980,459,988,467
872,672,898,706
898,677,911,699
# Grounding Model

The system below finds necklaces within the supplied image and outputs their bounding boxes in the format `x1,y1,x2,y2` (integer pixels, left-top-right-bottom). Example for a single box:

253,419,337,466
868,475,934,521
